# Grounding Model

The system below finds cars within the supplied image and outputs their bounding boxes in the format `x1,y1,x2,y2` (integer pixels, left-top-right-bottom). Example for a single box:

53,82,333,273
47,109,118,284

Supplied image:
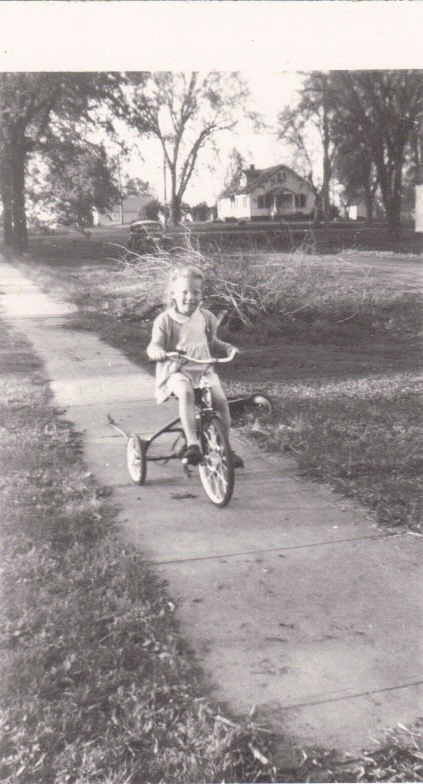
127,220,173,251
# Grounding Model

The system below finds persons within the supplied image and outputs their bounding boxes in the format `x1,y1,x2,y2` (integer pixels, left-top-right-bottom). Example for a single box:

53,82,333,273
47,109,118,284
145,265,245,470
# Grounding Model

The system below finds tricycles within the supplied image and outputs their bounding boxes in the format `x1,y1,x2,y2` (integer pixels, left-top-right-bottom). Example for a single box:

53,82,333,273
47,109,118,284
126,347,242,507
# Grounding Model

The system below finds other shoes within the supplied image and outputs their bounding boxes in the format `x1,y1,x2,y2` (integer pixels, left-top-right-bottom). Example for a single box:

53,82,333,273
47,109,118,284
183,444,202,466
222,451,243,468
